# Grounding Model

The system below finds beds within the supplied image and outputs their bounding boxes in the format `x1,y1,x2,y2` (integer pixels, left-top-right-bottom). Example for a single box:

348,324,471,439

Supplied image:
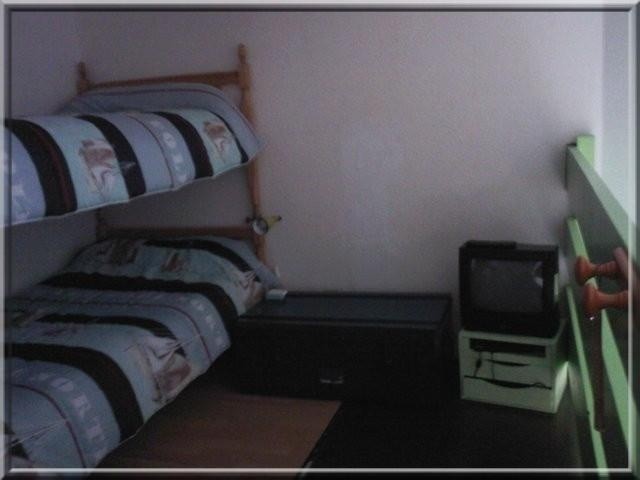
4,44,267,469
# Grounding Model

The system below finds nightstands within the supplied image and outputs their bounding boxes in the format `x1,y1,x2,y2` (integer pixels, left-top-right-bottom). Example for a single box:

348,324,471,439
237,293,452,412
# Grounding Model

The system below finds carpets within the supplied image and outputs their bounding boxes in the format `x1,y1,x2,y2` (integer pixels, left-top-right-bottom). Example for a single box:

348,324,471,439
294,401,594,480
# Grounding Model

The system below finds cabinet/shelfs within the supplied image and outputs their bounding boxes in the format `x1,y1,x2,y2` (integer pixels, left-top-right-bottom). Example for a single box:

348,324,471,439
458,319,569,414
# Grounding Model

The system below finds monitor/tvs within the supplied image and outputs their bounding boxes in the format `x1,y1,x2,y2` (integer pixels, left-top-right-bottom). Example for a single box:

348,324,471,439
460,240,560,339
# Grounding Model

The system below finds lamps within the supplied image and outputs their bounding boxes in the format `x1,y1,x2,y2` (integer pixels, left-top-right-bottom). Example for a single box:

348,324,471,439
253,216,290,305
